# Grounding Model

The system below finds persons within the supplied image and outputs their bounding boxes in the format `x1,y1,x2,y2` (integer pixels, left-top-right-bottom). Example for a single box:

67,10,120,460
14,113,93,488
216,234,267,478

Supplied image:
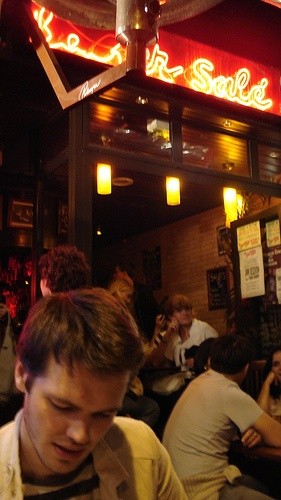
0,246,281,500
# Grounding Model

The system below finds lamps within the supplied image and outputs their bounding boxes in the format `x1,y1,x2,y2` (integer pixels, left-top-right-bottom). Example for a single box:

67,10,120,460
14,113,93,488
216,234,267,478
222,186,237,226
95,159,113,197
163,173,183,206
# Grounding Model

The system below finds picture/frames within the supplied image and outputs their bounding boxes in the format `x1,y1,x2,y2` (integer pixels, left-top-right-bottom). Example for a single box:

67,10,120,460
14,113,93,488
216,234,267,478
216,224,231,256
7,197,34,228
